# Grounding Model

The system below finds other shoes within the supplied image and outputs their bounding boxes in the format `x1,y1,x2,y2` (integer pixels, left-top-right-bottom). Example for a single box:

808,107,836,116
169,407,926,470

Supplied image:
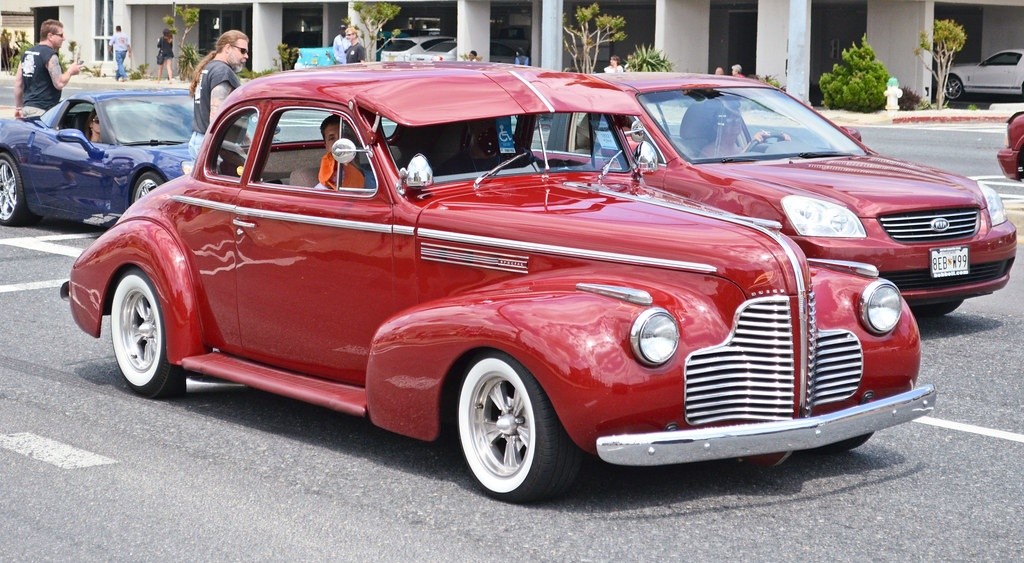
78,197,111,210
53,182,79,191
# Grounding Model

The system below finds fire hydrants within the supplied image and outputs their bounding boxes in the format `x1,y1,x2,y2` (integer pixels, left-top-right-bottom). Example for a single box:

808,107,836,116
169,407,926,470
882,76,904,111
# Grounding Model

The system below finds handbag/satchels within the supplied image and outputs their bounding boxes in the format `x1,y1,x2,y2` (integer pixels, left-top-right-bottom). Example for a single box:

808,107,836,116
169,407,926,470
157,49,164,64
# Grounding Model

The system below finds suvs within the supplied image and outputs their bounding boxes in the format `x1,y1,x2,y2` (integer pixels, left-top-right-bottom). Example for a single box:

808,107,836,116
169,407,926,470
379,34,457,61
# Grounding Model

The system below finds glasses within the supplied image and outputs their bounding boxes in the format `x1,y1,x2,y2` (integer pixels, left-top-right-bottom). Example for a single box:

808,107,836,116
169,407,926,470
91,118,100,123
52,33,63,37
230,44,248,54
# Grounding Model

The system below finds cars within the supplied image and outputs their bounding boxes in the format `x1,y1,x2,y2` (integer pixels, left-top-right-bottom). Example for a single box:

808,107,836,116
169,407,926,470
996,110,1024,182
943,47,1024,102
410,38,529,65
57,59,940,506
502,70,1020,319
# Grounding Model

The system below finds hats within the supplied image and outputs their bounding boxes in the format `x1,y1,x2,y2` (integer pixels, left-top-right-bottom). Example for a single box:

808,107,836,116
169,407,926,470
732,65,742,73
339,24,346,29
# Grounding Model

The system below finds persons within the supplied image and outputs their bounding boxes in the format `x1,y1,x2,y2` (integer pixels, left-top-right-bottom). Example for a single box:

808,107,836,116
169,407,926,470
85,108,100,142
604,55,623,73
109,25,131,82
333,24,351,64
715,65,725,74
312,114,377,189
698,104,791,158
731,64,746,78
469,51,478,62
344,28,365,64
514,48,529,66
154,28,174,84
14,19,83,118
188,29,250,167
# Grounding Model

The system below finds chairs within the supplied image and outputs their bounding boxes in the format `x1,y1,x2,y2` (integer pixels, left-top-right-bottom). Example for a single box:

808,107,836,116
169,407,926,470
76,111,91,132
290,165,322,188
682,103,721,160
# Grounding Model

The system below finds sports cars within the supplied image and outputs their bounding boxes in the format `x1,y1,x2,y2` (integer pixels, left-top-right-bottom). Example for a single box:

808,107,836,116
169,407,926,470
0,88,283,230
